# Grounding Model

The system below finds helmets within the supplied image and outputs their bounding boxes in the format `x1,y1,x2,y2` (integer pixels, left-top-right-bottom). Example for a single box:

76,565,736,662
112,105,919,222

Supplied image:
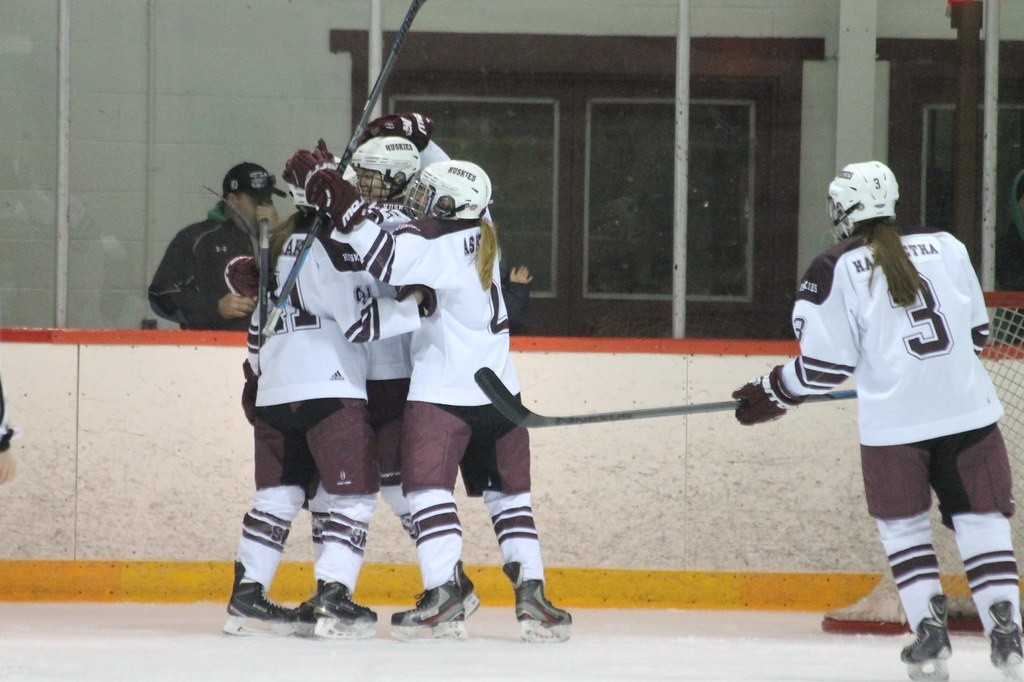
829,161,899,238
403,161,491,224
351,134,421,206
289,158,358,211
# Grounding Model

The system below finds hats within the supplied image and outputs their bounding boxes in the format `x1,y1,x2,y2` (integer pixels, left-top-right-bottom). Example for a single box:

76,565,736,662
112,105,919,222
221,156,288,199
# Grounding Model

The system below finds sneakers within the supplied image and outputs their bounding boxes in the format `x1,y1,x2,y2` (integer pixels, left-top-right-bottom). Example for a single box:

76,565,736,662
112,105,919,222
296,592,320,637
453,561,481,619
222,559,301,635
504,561,571,643
390,579,466,642
902,593,954,682
988,600,1023,679
313,575,378,639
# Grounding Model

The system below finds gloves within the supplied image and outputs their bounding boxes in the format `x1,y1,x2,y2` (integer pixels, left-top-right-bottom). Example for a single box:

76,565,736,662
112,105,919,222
303,174,363,236
242,375,257,427
362,111,435,151
396,283,436,318
280,140,333,187
732,362,804,425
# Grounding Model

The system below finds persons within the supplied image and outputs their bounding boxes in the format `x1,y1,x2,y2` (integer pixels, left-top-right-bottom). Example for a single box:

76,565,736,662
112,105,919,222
506,265,533,335
147,161,287,332
731,162,1024,682
223,112,573,642
0,372,16,486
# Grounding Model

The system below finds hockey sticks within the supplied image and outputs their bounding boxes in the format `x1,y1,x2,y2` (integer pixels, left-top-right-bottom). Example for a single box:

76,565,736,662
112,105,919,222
472,365,857,429
251,0,426,379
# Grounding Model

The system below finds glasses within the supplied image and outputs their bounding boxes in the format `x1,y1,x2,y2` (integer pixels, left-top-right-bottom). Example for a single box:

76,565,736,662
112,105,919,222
231,174,276,196
